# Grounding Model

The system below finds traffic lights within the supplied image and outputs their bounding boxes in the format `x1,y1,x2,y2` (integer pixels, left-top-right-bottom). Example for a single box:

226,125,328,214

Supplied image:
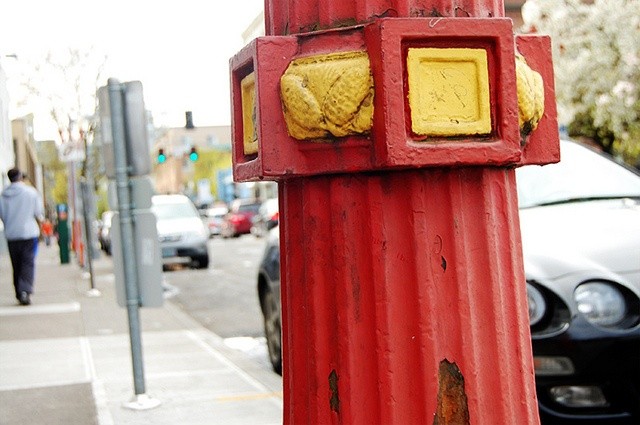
158,148,166,162
188,146,198,160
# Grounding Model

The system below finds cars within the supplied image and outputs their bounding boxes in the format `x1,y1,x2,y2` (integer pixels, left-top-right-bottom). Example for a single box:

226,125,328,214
250,200,279,236
228,211,257,236
258,138,640,424
205,206,229,235
99,211,111,255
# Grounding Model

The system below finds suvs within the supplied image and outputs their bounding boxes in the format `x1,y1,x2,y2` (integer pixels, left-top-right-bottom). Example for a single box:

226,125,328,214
150,193,208,269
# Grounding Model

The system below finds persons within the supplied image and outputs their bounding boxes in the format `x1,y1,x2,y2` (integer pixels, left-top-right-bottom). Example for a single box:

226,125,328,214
41,218,53,246
0,168,45,305
